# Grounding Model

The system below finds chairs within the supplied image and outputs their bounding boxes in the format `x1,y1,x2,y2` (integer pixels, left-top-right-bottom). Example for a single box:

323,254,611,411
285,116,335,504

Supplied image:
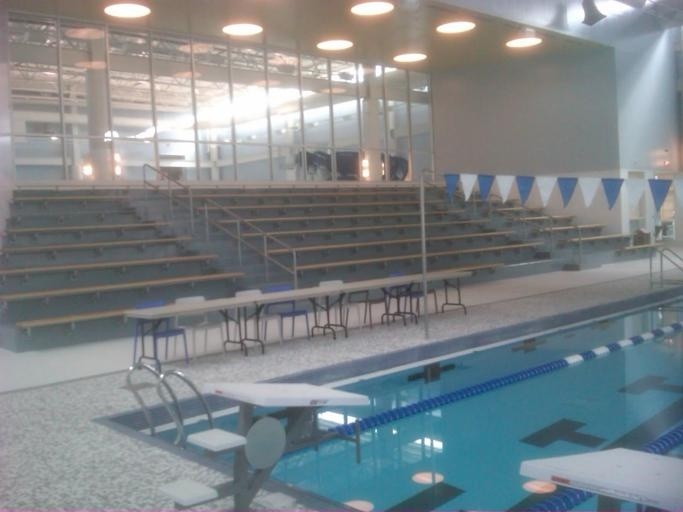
135,274,440,362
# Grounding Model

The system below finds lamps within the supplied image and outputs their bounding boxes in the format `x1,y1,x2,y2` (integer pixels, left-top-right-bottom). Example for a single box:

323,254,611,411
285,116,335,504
393,46,427,63
351,2,395,16
102,1,153,17
434,11,477,35
316,32,354,51
222,17,264,35
503,27,543,47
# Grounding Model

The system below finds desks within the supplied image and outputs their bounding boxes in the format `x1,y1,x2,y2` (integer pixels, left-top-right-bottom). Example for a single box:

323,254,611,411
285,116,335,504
321,270,473,336
124,298,254,372
234,286,340,352
0,185,666,341
